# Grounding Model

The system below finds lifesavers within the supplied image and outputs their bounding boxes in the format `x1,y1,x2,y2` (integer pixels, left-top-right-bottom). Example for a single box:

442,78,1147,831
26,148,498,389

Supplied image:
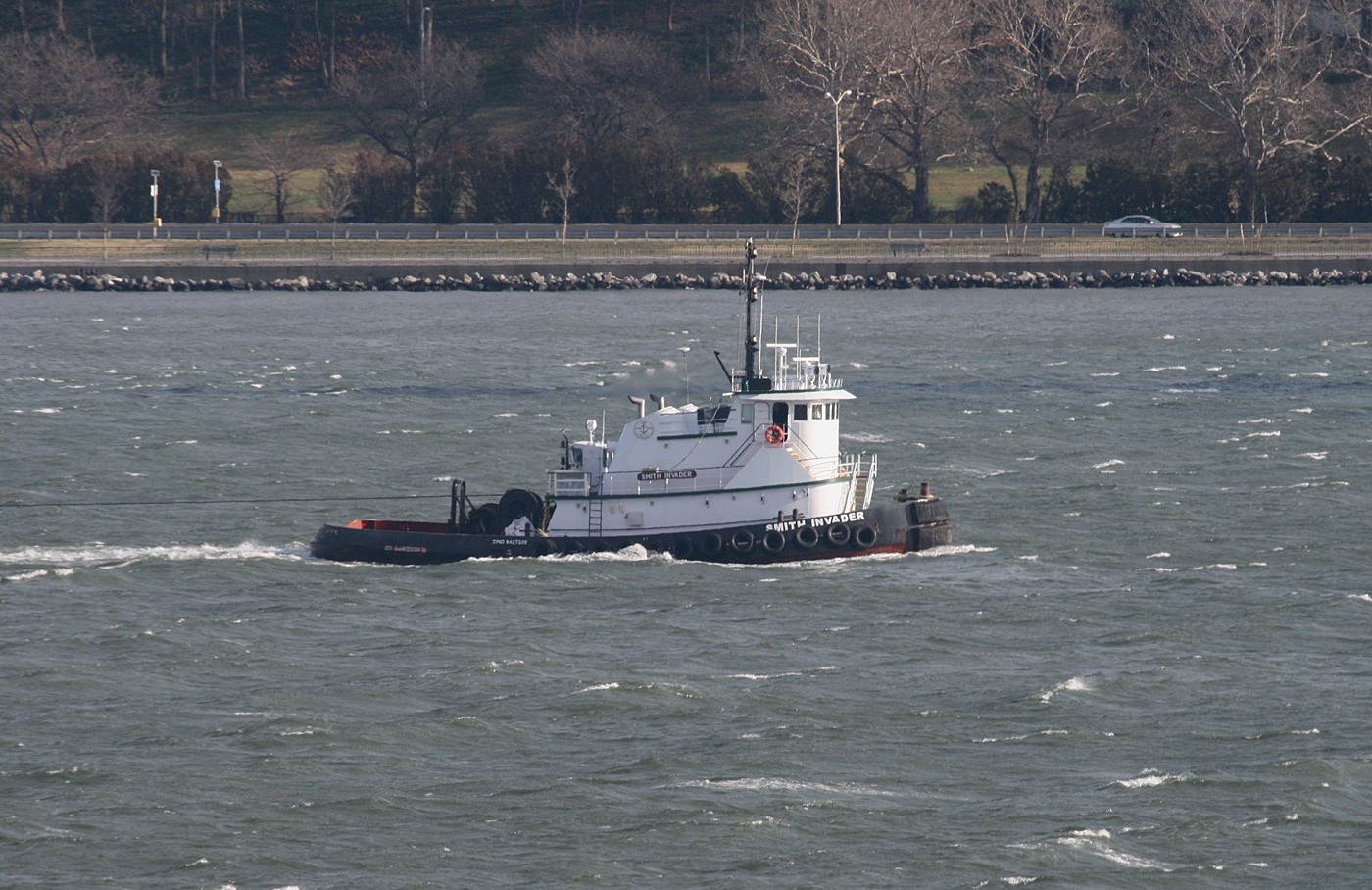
730,529,754,554
825,522,850,548
670,536,694,558
852,525,877,549
762,531,785,554
698,532,723,556
531,540,555,557
641,538,662,557
617,539,633,552
765,426,784,444
796,526,819,548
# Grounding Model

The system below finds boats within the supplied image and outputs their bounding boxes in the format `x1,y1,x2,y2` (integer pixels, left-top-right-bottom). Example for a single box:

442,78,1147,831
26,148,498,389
311,237,951,565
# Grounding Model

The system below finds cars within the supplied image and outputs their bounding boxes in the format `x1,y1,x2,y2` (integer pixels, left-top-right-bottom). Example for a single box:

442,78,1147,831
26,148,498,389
1102,214,1183,237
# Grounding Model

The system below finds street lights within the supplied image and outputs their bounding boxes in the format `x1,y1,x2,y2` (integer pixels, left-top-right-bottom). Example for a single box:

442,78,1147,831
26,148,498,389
151,169,160,239
824,89,852,225
212,159,223,222
